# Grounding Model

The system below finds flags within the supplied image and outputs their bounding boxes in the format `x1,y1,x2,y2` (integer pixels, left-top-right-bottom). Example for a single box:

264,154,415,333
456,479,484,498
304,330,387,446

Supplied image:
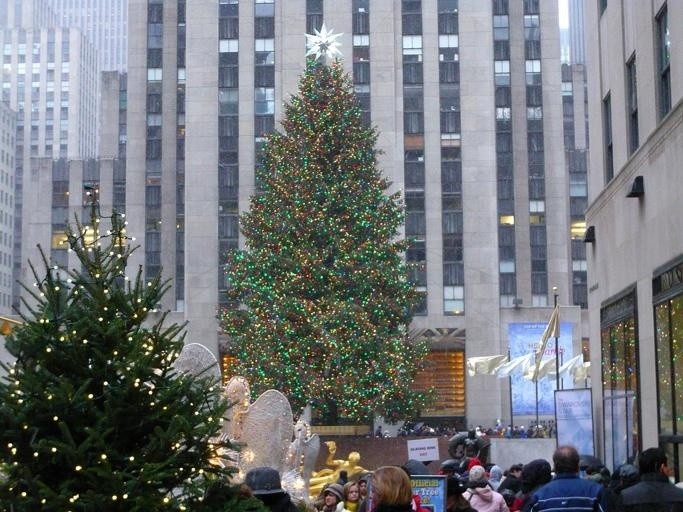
467,353,592,389
531,305,559,384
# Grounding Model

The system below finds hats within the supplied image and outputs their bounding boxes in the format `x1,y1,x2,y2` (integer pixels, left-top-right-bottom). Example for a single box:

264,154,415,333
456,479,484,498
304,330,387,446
324,482,345,501
468,465,487,484
245,467,286,497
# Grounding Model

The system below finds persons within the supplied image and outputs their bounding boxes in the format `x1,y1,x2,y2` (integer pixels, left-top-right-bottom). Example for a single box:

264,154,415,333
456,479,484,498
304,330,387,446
309,440,367,495
396,465,430,511
404,417,557,439
357,478,367,509
342,479,361,512
318,483,351,512
442,443,683,512
369,465,417,511
